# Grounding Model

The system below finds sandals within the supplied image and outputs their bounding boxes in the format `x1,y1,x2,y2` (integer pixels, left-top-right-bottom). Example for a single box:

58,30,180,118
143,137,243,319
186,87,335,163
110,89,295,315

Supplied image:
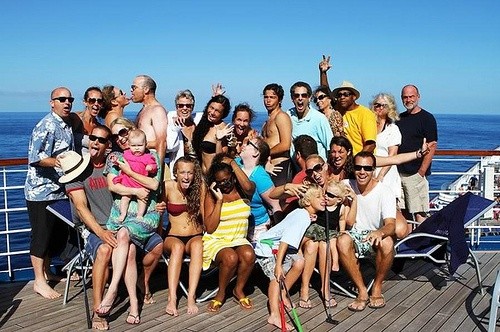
298,296,339,309
92,291,154,332
347,293,387,310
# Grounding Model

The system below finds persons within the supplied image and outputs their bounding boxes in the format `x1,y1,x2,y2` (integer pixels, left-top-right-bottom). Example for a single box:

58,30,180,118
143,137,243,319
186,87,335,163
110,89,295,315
163,83,226,230
370,93,402,198
319,54,377,155
24,87,76,299
98,85,137,133
261,83,292,186
131,74,168,165
394,85,439,234
286,82,334,171
65,87,104,156
156,135,438,331
65,118,166,330
310,85,345,136
191,95,235,174
229,103,283,177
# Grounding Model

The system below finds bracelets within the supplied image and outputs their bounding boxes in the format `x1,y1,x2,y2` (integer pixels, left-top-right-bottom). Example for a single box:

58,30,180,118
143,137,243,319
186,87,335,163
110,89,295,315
417,150,422,158
230,159,234,165
284,185,287,191
215,135,222,141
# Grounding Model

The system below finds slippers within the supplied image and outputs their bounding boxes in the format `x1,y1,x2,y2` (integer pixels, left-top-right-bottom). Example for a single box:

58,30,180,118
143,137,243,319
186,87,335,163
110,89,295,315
208,297,253,310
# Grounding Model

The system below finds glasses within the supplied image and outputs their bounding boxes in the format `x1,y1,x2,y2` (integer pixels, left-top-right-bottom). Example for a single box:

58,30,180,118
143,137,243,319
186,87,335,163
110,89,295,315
353,164,374,172
303,163,329,174
293,93,320,101
88,134,107,143
246,140,257,149
176,103,195,110
52,97,78,104
87,98,103,105
131,85,137,90
325,191,339,198
374,102,391,111
112,128,131,142
336,92,351,98
314,95,331,102
114,89,123,97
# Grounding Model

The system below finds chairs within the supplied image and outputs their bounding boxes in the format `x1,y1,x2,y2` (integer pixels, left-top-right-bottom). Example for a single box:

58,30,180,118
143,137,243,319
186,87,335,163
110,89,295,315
47,199,358,301
355,191,497,295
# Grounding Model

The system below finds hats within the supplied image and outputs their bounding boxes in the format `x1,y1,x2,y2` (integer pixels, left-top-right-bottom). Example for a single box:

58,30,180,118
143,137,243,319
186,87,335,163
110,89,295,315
56,150,93,184
332,82,362,99
